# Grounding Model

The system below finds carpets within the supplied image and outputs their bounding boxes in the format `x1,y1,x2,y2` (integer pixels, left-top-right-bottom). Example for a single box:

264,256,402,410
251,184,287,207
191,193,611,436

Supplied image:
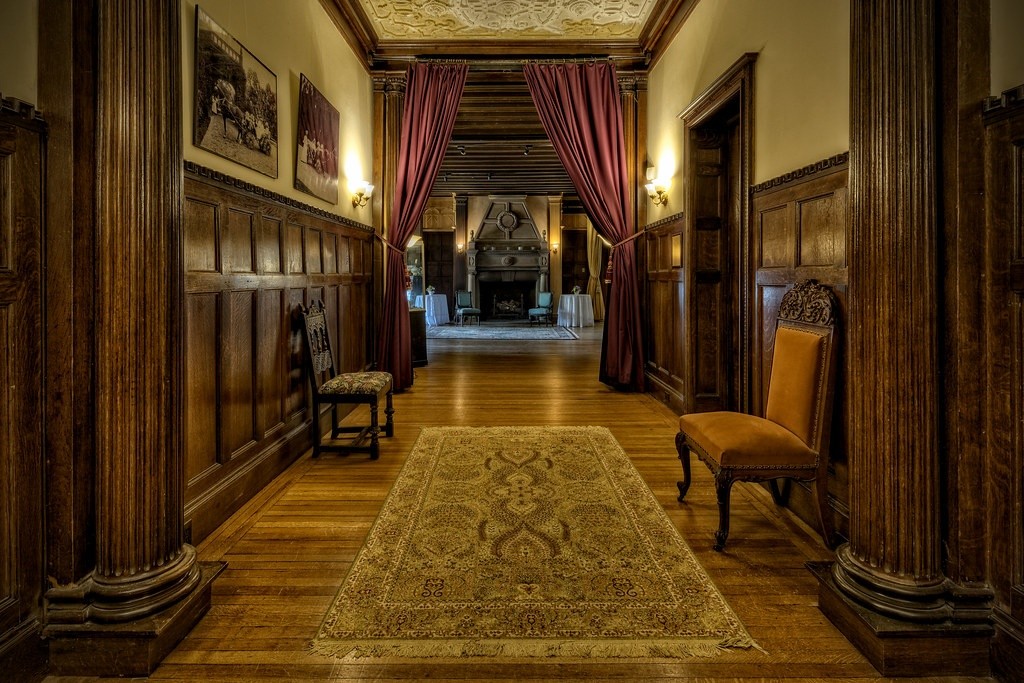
426,326,580,340
305,426,771,657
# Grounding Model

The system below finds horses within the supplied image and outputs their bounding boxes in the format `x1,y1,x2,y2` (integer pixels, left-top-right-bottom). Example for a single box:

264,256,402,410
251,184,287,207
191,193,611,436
212,81,245,144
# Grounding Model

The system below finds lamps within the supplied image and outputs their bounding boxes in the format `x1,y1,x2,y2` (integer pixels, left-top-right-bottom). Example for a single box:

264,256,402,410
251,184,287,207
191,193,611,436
553,244,559,254
352,181,374,208
457,244,464,254
645,179,668,206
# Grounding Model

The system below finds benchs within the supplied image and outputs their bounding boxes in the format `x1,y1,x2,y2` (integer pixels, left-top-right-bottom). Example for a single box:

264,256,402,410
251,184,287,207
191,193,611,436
676,279,842,551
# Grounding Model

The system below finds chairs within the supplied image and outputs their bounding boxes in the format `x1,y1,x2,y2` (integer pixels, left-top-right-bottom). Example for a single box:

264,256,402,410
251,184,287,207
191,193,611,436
455,290,481,327
297,299,396,457
529,291,553,327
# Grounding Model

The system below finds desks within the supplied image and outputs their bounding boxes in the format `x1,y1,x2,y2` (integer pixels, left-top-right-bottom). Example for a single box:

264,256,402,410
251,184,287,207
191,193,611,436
415,294,450,325
557,294,595,326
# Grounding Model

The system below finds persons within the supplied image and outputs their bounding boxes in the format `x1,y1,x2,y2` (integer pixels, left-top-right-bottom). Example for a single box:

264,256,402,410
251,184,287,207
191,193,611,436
301,130,309,163
310,130,326,177
